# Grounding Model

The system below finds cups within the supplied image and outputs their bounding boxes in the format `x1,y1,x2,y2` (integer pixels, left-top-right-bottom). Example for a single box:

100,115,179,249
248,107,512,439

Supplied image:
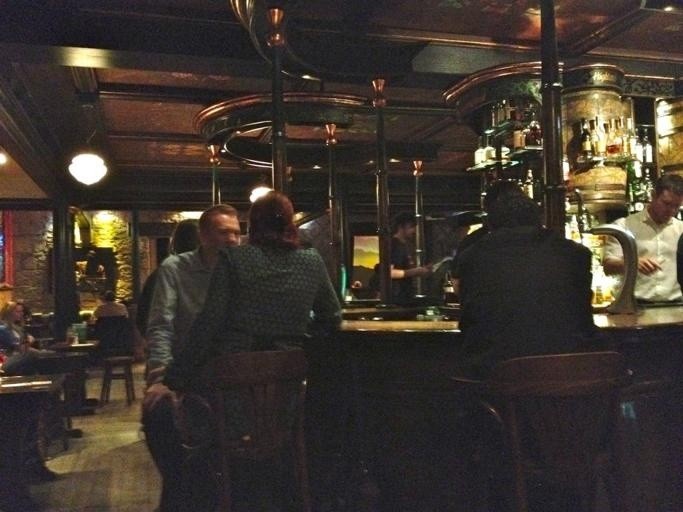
425,305,441,317
341,289,352,305
65,327,81,345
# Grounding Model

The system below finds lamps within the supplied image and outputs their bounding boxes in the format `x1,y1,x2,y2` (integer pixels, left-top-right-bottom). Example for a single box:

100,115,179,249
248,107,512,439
65,128,107,187
247,171,273,205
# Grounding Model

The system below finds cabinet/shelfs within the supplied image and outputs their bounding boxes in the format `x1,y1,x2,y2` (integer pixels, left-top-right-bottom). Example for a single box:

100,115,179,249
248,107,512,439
440,61,566,219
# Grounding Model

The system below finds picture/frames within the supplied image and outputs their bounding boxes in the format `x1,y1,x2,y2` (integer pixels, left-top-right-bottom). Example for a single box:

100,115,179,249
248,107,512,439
0,207,14,291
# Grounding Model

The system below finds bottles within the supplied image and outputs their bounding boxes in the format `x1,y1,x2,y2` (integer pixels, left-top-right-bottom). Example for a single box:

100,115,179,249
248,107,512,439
565,115,656,304
19,330,29,355
473,103,544,207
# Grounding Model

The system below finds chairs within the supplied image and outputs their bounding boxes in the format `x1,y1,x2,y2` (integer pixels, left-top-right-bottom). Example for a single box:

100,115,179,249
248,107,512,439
445,347,663,512
169,345,321,512
